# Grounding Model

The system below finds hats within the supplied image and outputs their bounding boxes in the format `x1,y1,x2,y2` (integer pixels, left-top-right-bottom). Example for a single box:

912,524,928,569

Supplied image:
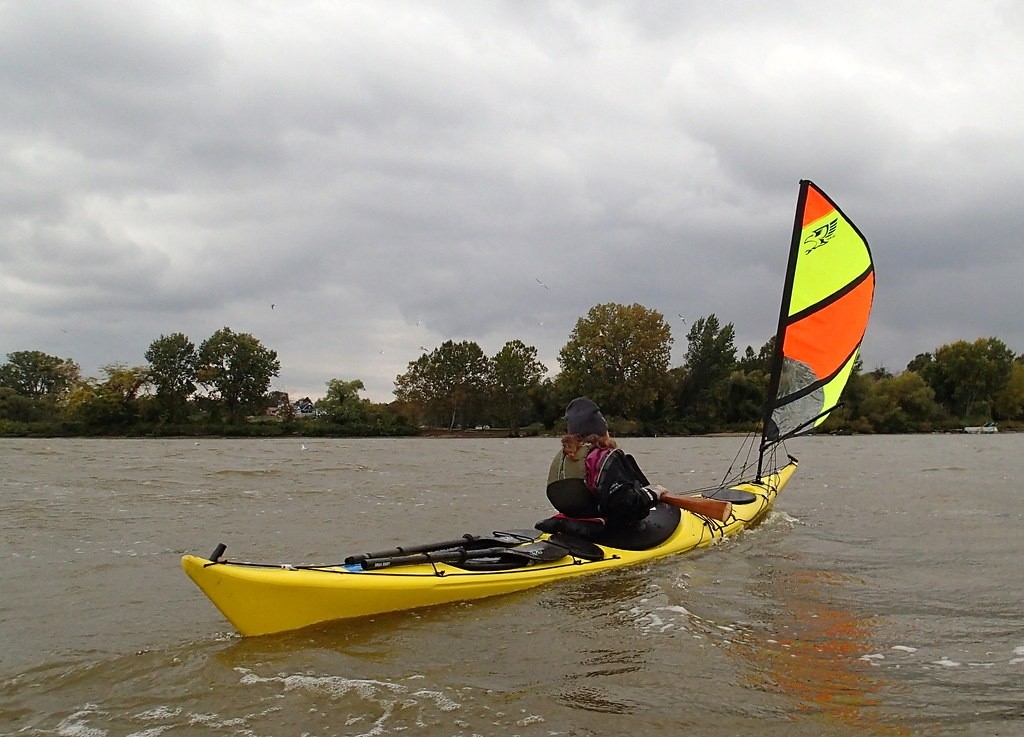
564,397,609,437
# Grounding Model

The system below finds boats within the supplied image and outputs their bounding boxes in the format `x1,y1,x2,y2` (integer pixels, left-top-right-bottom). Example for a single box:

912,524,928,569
932,422,1000,435
180,175,878,638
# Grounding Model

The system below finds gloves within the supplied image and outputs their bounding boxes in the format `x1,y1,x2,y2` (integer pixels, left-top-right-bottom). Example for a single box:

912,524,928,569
653,485,668,500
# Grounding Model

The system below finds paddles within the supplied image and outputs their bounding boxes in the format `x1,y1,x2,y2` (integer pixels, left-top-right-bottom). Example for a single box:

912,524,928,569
362,541,569,566
652,484,732,522
343,529,545,562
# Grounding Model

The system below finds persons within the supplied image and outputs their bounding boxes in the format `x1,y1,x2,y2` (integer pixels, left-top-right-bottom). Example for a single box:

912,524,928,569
548,399,670,524
